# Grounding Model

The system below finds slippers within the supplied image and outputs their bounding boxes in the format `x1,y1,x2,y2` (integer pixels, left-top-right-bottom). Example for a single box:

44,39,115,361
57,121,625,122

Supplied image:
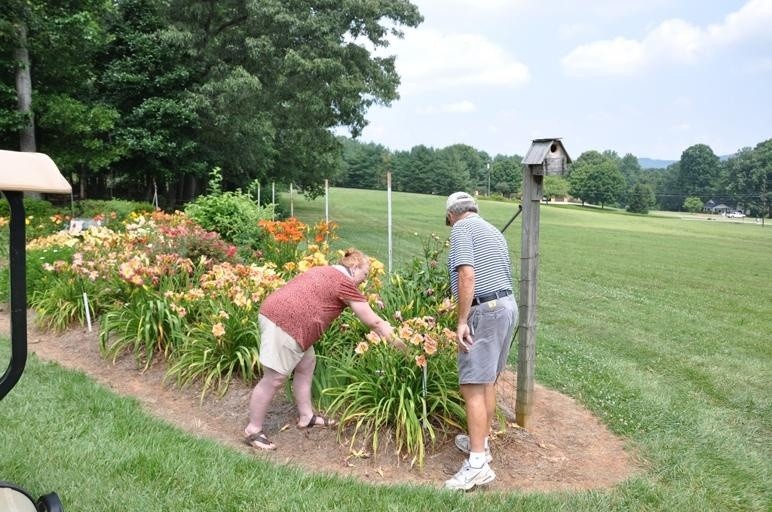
296,413,339,430
238,430,276,451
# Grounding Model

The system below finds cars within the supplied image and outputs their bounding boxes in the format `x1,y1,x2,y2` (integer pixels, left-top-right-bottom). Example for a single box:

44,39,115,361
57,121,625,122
542,193,553,204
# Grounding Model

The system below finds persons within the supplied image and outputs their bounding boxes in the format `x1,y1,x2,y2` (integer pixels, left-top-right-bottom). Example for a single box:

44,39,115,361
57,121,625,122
239,247,407,452
444,190,520,492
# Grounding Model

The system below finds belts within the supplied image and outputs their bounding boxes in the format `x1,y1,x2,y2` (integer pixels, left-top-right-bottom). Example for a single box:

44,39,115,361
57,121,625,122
471,289,512,307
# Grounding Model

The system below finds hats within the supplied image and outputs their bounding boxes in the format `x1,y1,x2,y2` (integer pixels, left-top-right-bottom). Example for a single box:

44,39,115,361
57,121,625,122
445,192,476,226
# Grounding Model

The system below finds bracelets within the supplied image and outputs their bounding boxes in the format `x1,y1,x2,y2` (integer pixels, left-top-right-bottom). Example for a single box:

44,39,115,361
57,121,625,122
386,334,397,344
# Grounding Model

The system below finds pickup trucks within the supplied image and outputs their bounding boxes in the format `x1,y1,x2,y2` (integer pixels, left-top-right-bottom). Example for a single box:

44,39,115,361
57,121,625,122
727,211,746,219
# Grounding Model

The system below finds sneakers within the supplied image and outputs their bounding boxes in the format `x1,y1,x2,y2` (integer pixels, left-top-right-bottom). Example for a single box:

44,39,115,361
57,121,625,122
446,434,496,491
